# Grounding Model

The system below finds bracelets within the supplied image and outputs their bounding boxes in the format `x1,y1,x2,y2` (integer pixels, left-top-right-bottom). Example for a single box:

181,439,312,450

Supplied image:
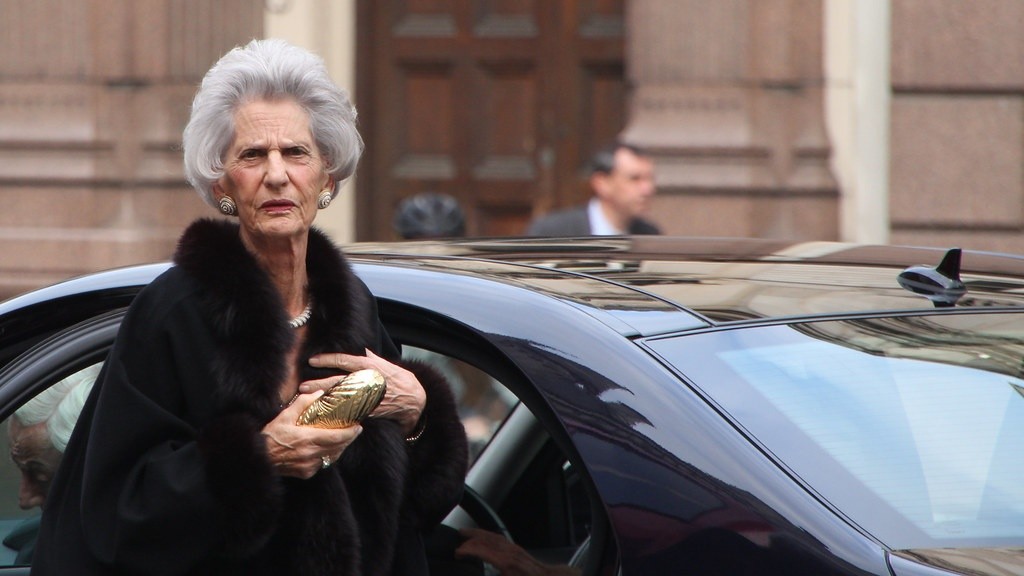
405,415,428,447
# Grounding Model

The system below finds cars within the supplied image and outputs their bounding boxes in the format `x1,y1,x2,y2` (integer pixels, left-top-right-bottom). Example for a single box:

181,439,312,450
0,234,1024,576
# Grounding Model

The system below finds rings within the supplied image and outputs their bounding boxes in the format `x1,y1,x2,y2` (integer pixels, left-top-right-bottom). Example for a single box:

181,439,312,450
322,457,330,468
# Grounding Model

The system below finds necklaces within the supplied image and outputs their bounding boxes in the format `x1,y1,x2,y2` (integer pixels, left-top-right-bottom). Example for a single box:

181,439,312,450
284,293,312,330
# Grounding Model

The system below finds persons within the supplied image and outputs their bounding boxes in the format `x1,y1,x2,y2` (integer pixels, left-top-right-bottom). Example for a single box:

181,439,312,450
391,192,466,241
9,359,108,514
24,37,469,576
525,141,661,239
